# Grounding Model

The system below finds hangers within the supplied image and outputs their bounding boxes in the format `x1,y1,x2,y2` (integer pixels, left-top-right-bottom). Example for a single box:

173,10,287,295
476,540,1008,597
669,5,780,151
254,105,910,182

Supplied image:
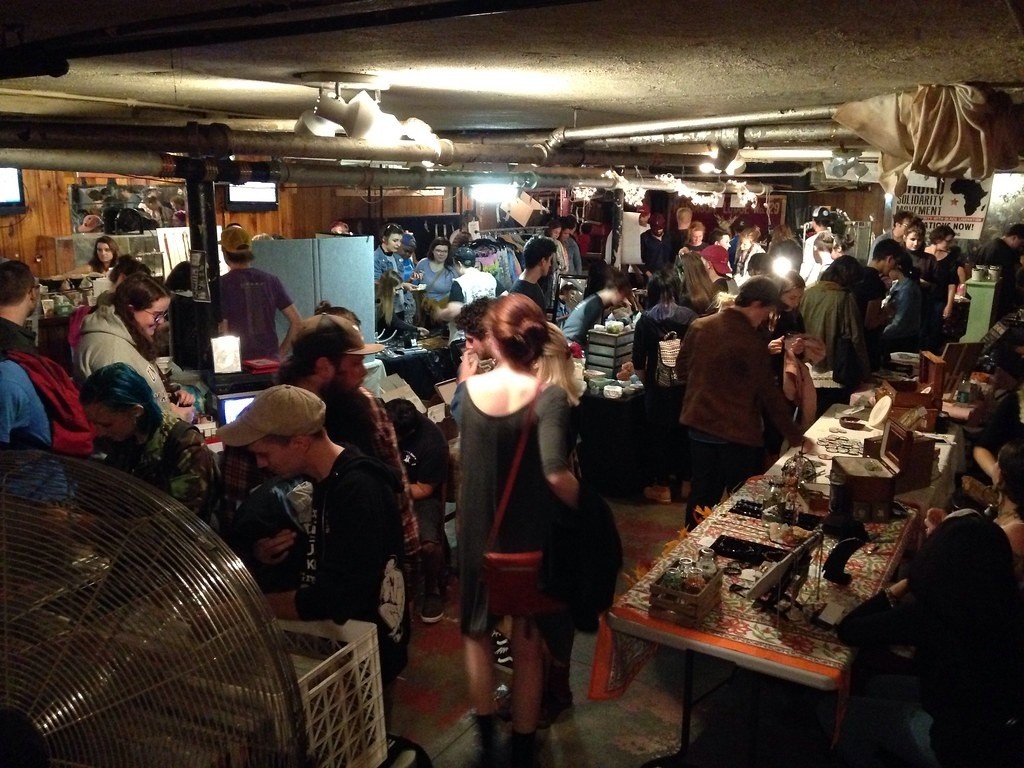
463,231,529,254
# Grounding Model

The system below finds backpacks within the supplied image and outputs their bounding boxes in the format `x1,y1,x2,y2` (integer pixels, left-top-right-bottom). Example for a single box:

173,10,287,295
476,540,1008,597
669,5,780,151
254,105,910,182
642,309,694,387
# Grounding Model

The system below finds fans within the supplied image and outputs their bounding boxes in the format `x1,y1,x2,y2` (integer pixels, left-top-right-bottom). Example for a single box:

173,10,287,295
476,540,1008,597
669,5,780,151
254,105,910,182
1,448,310,768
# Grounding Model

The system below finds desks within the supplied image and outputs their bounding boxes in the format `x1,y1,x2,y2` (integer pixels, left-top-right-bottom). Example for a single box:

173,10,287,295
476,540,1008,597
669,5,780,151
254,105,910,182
577,389,652,503
586,402,967,768
375,331,465,401
855,370,995,438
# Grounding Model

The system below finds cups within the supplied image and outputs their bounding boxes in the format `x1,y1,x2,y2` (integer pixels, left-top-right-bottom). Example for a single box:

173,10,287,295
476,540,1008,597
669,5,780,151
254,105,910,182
40,286,97,319
972,264,1002,282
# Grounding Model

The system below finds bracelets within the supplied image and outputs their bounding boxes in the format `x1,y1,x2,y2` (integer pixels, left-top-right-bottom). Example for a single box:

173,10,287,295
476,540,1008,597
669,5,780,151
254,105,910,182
864,461,883,472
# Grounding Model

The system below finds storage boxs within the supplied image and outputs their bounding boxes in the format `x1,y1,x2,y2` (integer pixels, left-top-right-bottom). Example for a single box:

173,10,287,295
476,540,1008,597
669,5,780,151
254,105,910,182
82,600,388,768
648,559,724,629
584,327,634,380
828,350,947,524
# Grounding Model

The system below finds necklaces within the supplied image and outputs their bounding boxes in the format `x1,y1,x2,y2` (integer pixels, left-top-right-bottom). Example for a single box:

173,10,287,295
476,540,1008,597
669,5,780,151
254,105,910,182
709,514,771,542
762,551,787,562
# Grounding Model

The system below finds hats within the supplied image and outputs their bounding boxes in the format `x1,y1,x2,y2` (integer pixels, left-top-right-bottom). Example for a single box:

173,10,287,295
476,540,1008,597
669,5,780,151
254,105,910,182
291,312,386,355
812,206,831,217
218,227,252,253
216,385,326,446
694,245,731,274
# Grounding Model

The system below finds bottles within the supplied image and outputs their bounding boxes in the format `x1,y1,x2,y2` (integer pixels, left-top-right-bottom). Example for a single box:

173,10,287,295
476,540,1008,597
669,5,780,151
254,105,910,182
660,547,716,605
760,476,785,528
957,374,971,405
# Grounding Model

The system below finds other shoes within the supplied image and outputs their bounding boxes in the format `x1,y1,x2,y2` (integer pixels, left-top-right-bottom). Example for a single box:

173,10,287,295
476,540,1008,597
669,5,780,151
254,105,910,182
420,591,444,622
644,482,673,505
494,692,514,719
537,693,573,728
681,481,690,499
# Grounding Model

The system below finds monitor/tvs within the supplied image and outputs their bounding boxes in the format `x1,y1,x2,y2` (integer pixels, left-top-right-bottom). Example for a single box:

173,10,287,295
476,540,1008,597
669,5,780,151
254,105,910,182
0,168,25,215
216,391,255,427
223,182,278,212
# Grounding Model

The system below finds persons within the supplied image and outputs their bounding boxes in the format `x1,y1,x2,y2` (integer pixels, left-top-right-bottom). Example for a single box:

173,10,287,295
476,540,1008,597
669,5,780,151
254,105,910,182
0,207,1024,419
950,346,1024,508
384,399,452,624
76,366,226,611
453,297,626,725
631,278,701,504
585,187,616,261
254,312,423,607
765,270,805,456
923,437,1024,583
215,384,412,736
457,293,584,767
0,356,69,581
839,512,1024,768
68,183,186,236
674,281,812,533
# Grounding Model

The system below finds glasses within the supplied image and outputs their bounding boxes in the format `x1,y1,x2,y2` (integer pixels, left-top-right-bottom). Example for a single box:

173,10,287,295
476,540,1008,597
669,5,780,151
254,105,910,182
142,309,169,325
28,284,40,297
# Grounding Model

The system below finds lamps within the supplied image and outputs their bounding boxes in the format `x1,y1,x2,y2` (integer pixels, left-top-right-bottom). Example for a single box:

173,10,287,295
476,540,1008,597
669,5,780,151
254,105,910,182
825,148,870,179
294,70,433,141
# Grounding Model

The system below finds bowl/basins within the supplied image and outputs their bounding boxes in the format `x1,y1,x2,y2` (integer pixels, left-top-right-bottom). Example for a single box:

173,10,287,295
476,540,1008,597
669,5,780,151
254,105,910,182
839,416,865,430
594,321,624,331
583,362,644,399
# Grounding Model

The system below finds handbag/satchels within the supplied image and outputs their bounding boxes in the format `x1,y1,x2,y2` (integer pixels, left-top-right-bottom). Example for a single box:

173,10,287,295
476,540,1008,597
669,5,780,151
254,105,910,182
832,336,865,387
483,552,567,617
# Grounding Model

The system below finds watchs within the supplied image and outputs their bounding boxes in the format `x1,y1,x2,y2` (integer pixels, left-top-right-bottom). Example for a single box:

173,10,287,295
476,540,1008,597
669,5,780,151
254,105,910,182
884,587,896,607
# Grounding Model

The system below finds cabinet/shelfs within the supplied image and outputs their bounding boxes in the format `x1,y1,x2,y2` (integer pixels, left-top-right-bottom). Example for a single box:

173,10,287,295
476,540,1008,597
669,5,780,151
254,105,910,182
25,315,75,369
35,231,164,281
